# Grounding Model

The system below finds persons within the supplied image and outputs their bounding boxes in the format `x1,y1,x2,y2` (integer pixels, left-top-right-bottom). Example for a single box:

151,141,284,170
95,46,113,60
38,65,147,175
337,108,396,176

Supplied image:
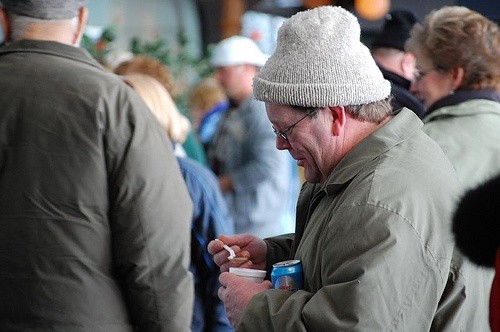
0,0,197,332
101,6,500,332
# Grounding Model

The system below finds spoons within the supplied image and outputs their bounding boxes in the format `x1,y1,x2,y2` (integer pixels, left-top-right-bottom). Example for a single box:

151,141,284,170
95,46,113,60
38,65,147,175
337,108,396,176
214,238,248,265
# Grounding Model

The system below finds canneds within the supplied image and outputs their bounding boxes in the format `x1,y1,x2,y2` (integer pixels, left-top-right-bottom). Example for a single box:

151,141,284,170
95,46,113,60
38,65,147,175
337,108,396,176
270,260,305,292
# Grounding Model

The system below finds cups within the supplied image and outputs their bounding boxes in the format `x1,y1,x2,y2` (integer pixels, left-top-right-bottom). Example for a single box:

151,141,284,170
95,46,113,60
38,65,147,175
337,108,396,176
229,267,267,283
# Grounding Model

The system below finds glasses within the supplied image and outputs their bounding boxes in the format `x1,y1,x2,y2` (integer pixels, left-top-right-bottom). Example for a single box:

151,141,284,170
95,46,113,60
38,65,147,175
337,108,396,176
272,108,316,142
413,66,436,81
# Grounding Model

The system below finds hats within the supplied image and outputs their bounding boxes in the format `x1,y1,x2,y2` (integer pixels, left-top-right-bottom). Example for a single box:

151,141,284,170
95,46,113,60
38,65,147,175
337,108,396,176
253,6,391,108
211,36,268,67
103,49,134,72
0,0,86,19
371,8,422,53
